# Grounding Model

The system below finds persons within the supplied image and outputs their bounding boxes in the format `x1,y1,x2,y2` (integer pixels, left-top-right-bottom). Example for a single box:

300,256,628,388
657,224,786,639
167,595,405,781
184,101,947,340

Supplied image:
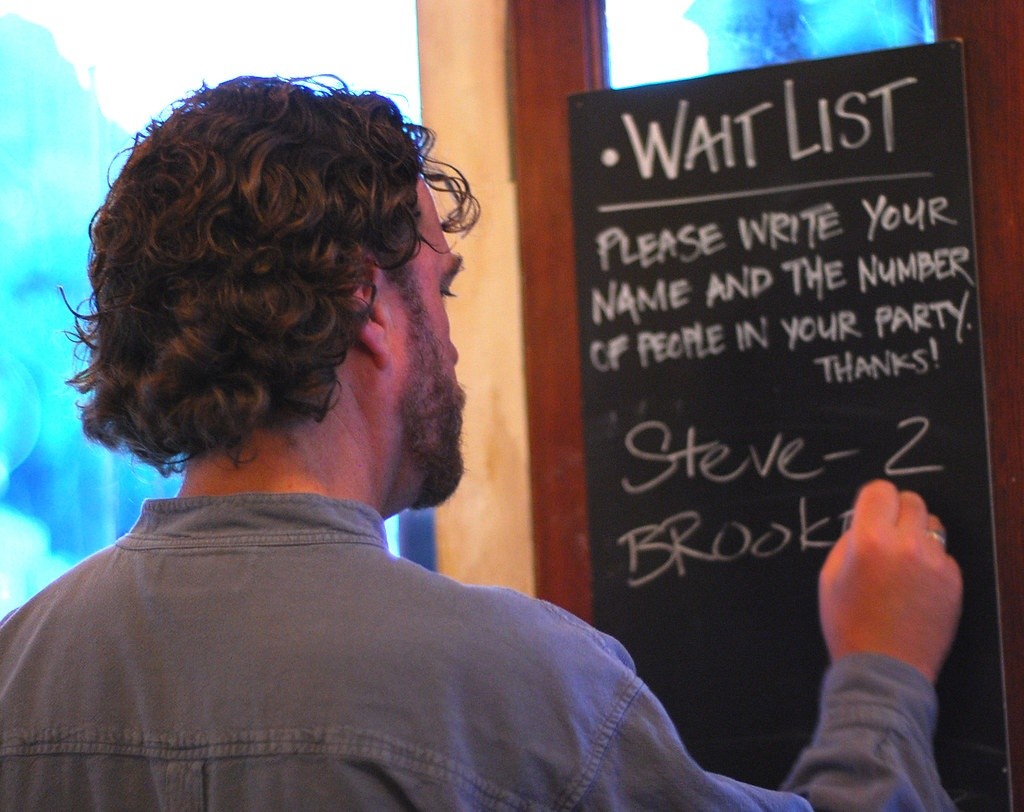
0,73,963,812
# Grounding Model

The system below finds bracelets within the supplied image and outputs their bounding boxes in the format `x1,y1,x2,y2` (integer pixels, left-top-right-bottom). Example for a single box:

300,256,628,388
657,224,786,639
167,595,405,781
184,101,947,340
925,530,946,543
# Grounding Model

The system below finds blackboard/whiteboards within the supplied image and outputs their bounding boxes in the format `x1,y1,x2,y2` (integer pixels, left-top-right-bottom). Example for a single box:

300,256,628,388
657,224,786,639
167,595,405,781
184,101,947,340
569,33,1012,812
